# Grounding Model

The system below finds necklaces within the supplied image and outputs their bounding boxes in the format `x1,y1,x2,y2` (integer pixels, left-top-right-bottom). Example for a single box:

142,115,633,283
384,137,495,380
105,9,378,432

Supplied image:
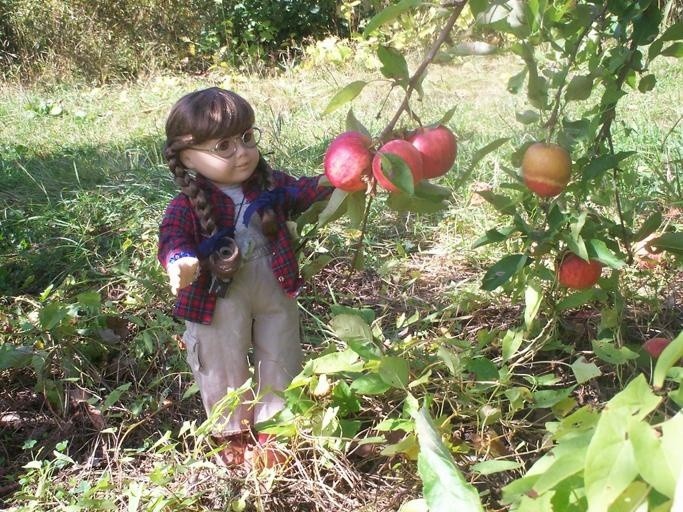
233,196,246,230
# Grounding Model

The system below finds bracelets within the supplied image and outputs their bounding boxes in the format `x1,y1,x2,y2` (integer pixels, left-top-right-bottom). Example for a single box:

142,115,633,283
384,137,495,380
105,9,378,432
167,252,194,265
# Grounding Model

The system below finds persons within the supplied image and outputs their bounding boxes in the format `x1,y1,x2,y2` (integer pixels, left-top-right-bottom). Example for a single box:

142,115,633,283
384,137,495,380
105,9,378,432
153,86,338,475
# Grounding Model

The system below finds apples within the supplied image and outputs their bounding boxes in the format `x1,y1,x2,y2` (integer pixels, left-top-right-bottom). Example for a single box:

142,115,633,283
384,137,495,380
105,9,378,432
372,138,422,193
410,125,457,179
522,142,571,198
560,253,602,288
641,337,669,357
325,131,378,191
